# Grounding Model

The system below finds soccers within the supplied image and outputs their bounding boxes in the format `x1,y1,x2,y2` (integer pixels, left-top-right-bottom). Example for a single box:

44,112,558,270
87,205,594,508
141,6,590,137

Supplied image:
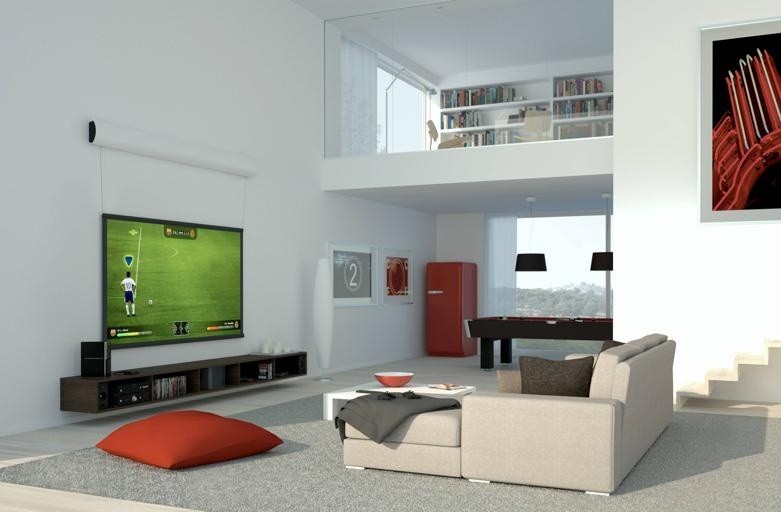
148,300,153,305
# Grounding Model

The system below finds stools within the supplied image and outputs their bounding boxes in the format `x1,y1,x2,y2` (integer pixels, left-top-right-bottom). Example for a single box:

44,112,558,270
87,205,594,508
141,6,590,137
344,407,461,478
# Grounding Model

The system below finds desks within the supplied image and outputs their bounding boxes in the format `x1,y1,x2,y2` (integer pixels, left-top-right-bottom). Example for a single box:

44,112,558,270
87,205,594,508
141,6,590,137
467,316,612,369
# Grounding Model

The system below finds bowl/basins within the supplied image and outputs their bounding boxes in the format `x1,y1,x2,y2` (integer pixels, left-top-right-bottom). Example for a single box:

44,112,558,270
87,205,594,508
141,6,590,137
374,373,414,387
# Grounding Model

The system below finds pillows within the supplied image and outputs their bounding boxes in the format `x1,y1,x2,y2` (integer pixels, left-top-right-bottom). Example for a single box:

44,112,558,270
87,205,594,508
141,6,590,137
594,340,627,369
518,356,594,397
95,410,284,469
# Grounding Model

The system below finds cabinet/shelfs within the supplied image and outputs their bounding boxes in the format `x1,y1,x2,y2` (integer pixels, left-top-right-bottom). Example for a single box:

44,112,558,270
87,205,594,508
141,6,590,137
440,70,614,149
60,352,306,414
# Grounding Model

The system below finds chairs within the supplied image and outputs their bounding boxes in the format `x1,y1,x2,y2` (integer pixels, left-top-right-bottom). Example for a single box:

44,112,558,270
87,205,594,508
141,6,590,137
513,111,552,141
426,120,469,150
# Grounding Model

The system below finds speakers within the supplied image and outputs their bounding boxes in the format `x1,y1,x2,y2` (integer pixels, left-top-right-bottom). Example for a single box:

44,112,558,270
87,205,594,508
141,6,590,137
81,340,112,378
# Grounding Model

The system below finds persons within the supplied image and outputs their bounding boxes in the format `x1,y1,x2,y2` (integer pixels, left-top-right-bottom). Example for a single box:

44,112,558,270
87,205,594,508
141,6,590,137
120,272,137,317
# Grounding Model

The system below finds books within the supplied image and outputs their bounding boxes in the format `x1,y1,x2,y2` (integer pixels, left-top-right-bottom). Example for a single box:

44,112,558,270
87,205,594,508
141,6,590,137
153,375,186,400
441,85,525,147
556,80,613,140
258,362,272,380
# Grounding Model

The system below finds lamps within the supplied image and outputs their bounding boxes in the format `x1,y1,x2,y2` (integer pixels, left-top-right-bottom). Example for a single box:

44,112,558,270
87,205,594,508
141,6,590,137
590,193,613,270
515,197,547,271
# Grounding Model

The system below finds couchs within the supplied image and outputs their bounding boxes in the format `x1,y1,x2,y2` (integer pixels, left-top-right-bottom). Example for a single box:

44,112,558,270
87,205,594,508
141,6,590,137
461,333,676,496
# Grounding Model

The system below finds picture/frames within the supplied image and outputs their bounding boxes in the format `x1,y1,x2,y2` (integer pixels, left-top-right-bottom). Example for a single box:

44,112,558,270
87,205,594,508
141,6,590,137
326,242,377,305
698,16,781,223
382,247,413,304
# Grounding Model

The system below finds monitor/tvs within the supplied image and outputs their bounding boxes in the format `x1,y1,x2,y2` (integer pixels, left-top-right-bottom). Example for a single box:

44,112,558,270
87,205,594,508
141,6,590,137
101,212,245,350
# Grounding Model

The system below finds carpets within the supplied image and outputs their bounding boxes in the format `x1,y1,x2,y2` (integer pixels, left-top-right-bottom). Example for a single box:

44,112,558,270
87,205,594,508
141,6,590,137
0,393,781,512
